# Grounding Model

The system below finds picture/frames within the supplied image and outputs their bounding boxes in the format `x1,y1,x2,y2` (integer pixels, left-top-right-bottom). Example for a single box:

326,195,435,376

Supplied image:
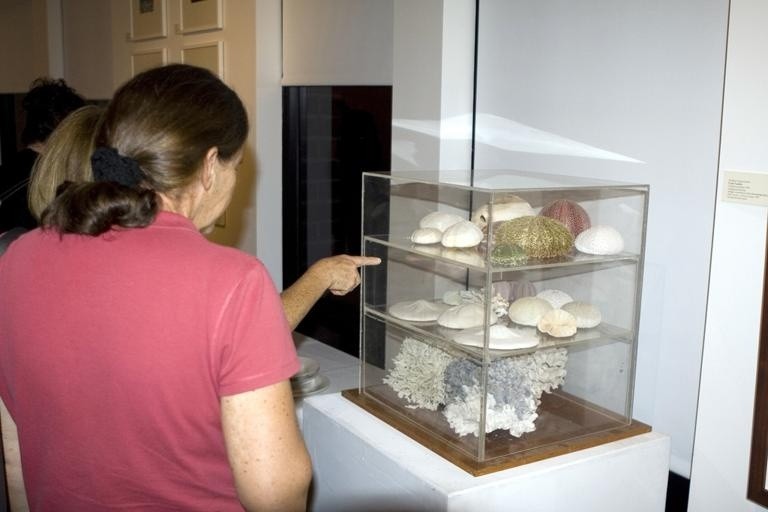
127,48,166,80
126,0,166,42
181,40,231,232
179,0,223,36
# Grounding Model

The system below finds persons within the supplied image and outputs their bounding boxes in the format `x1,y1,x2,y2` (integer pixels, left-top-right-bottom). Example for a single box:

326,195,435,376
1,63,315,512
0,99,382,340
0,74,88,234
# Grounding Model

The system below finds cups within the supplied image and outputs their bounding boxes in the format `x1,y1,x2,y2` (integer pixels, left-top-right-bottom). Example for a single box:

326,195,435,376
287,351,321,392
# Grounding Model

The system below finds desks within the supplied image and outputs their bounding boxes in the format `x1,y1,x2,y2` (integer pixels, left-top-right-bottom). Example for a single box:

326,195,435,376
296,385,671,511
282,326,394,445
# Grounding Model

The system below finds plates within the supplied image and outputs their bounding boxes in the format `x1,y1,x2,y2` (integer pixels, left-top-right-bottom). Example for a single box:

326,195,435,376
294,374,331,400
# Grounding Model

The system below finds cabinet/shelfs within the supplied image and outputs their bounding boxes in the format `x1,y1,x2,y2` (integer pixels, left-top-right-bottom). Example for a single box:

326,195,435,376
358,168,650,478
